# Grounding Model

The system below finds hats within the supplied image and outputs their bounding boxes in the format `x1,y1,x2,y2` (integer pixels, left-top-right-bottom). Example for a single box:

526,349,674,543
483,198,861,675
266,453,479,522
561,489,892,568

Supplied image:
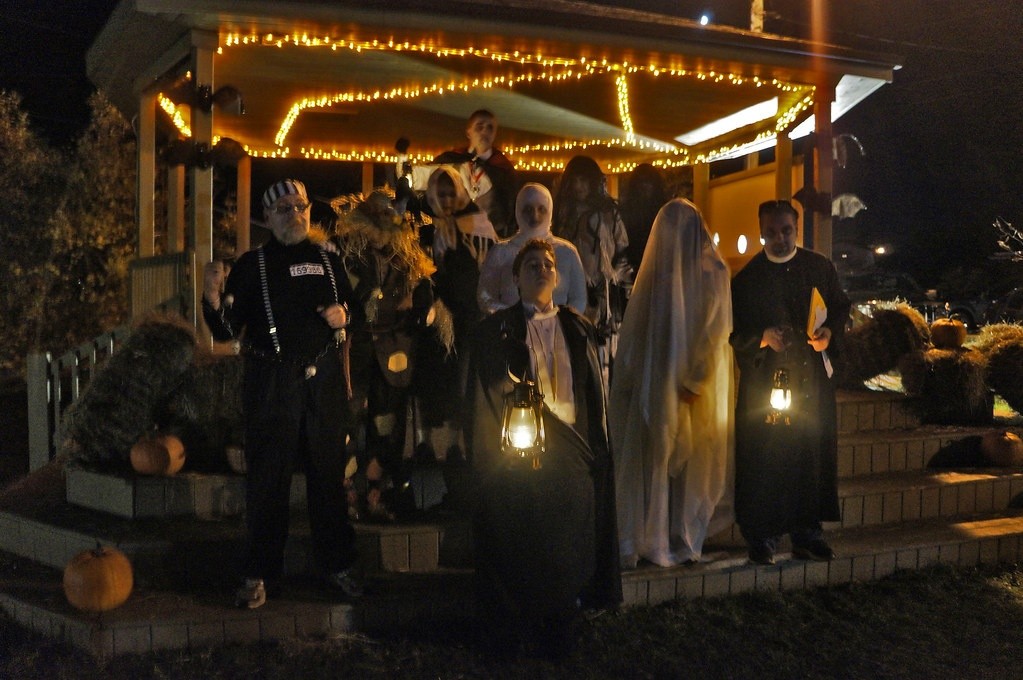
262,179,306,210
350,190,407,231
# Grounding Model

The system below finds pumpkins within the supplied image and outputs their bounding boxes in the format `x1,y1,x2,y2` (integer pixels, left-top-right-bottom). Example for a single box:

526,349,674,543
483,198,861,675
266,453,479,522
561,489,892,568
981,428,1023,468
931,314,966,348
63,539,134,611
130,424,185,477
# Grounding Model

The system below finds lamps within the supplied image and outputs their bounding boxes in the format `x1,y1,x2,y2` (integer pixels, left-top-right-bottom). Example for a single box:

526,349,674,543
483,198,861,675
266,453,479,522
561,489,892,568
498,342,546,469
765,340,794,428
200,85,244,117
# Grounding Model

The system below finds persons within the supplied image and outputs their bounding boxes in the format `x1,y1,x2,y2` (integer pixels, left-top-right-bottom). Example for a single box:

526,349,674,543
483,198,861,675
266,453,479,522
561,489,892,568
476,183,588,315
552,154,630,306
440,240,624,657
331,192,435,523
417,163,499,461
607,196,734,562
728,199,850,564
436,109,520,237
619,163,667,256
201,179,367,609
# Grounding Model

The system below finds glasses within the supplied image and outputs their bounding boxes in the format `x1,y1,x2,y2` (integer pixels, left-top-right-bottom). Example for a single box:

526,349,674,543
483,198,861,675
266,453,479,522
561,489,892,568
270,201,313,216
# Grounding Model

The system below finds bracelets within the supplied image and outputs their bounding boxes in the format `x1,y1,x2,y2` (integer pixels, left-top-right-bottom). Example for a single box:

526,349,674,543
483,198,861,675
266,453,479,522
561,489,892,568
209,296,220,304
342,304,350,324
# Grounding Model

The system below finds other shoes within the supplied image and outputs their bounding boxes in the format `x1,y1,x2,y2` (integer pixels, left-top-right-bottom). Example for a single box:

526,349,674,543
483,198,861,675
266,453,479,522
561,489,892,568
330,561,368,597
792,539,835,562
747,542,774,567
235,571,283,610
